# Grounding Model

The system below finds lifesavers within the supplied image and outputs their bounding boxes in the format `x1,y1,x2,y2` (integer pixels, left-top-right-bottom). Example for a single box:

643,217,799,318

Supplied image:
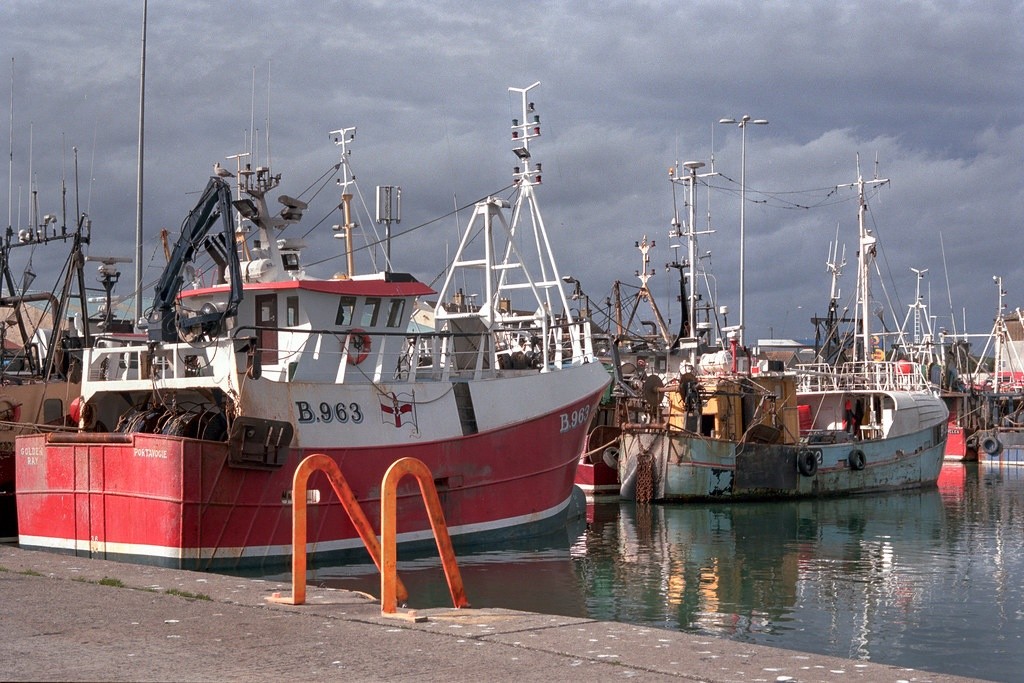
871,336,880,345
342,328,371,365
0,395,21,431
849,449,867,471
982,437,999,455
966,436,979,453
798,452,818,477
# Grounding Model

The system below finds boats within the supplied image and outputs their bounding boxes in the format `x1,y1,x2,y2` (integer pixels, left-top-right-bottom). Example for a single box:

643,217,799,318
938,272,1024,468
0,0,616,573
613,114,951,506
